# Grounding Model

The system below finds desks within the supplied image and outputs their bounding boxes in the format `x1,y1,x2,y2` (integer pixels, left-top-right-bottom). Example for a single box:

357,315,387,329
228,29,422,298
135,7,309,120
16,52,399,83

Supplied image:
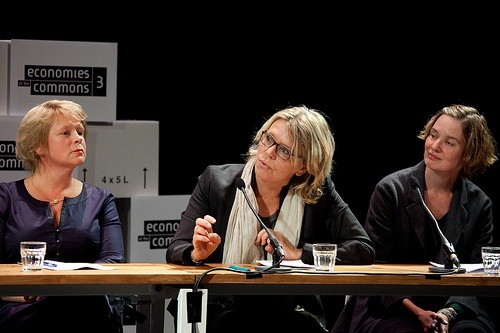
0,263,500,333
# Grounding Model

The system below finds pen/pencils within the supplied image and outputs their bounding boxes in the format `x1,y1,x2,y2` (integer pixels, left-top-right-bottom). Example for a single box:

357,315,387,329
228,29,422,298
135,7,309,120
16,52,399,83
229,265,250,271
43,262,57,267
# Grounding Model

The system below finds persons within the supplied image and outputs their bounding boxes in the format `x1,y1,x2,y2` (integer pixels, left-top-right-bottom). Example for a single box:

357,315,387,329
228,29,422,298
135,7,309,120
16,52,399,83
0,100,126,333
331,104,500,333
167,106,377,333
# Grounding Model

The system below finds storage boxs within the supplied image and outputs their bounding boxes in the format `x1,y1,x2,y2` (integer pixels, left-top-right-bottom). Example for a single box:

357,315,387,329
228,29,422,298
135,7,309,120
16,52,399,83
0,38,193,267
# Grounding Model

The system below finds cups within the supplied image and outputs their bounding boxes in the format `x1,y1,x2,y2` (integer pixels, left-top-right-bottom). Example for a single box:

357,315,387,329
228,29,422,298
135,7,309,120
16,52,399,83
481,246,500,274
20,241,46,272
312,243,337,271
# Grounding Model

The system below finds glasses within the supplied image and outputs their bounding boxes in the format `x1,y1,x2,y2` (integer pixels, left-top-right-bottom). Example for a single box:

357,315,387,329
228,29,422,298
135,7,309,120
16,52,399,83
259,131,304,161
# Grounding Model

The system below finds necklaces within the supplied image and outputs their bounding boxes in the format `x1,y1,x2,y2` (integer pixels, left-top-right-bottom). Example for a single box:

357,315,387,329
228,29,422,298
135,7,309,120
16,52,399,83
252,182,279,199
29,175,74,203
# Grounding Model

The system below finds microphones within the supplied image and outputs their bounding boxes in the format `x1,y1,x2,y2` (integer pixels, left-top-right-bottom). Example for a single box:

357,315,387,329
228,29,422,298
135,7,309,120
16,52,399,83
408,175,466,273
235,177,292,274
